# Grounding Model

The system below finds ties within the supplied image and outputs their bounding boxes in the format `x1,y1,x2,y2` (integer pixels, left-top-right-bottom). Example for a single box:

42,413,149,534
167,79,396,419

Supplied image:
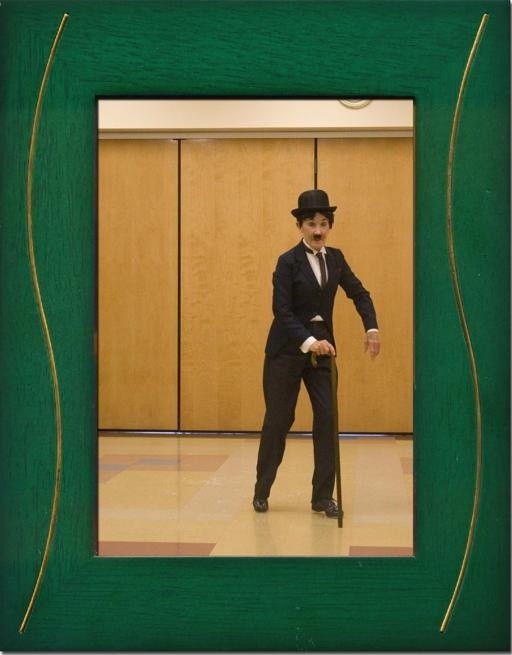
316,252,326,290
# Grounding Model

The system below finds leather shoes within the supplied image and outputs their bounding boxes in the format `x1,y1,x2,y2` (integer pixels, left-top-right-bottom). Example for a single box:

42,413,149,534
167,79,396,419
253,497,268,512
312,502,343,517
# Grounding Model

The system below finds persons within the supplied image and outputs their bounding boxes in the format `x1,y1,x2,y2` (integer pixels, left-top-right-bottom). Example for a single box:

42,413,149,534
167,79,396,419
253,190,381,517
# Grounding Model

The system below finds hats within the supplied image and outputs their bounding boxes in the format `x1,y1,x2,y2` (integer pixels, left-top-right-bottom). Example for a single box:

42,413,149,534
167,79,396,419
291,190,337,217
